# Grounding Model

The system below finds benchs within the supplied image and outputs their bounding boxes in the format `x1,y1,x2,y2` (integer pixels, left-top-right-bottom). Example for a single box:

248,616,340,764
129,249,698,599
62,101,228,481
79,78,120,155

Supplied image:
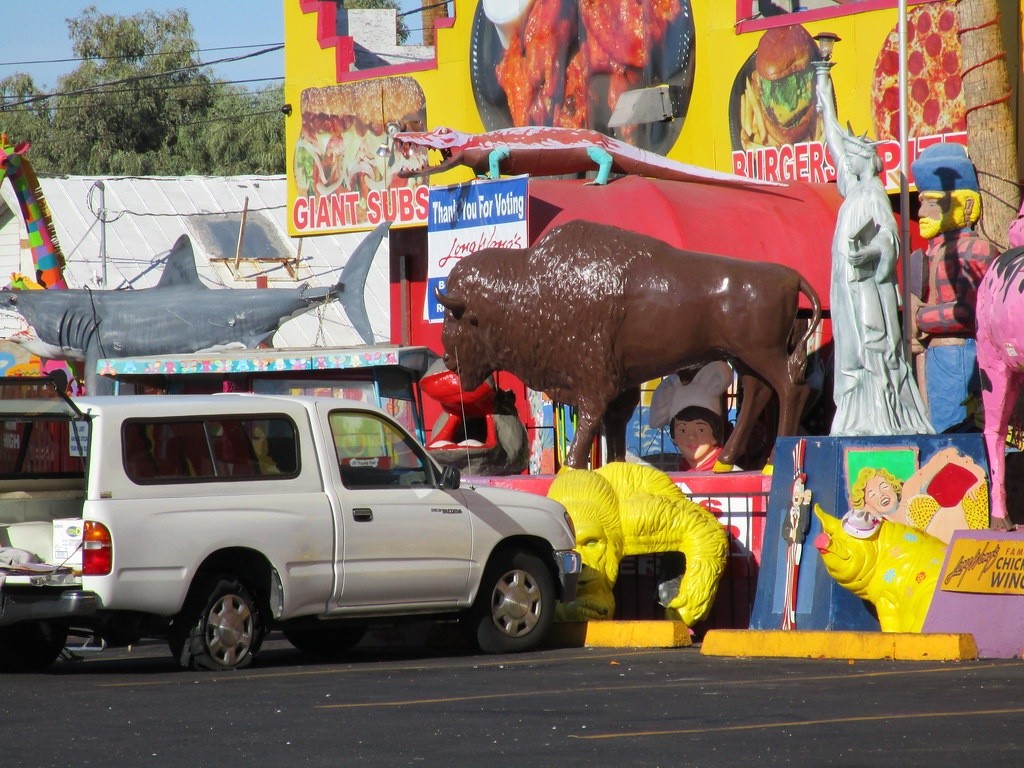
0,478,87,524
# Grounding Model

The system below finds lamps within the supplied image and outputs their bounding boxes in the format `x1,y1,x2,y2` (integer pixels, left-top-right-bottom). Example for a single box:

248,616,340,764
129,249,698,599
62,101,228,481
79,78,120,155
608,86,673,128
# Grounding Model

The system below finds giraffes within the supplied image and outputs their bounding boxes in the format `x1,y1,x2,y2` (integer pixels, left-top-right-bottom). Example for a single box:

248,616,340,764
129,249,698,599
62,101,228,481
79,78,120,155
1,133,80,398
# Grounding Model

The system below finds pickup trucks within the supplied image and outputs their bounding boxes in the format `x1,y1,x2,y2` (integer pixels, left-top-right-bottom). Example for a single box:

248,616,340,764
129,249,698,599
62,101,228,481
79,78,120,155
0,370,581,670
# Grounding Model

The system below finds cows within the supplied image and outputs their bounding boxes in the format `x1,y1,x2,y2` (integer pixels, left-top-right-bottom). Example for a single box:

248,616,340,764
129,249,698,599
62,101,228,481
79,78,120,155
434,220,823,475
